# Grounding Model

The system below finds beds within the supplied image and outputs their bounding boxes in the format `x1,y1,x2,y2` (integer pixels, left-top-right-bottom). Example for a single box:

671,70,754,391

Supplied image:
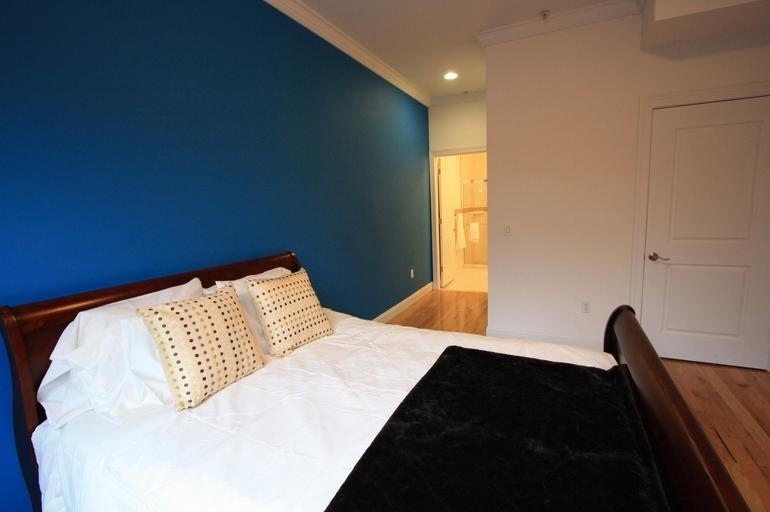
0,251,749,512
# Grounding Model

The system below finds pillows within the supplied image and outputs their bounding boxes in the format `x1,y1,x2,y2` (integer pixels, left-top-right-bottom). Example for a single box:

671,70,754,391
35,275,206,421
243,267,334,359
134,280,267,413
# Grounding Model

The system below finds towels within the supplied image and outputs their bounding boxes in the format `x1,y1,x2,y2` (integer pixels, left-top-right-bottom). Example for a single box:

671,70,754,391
469,222,480,243
455,212,467,250
